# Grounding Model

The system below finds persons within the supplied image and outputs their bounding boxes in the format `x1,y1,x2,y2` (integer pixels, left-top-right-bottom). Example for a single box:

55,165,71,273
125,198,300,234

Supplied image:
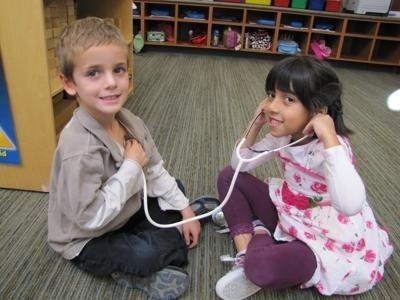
212,54,393,300
43,16,221,299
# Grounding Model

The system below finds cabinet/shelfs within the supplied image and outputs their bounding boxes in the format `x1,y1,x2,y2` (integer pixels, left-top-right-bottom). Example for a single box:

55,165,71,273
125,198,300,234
133,0,400,69
1,2,134,194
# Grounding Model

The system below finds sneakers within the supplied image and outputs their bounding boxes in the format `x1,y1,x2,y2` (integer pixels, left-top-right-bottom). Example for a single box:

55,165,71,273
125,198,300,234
213,209,230,233
189,196,220,223
215,254,262,300
113,265,189,299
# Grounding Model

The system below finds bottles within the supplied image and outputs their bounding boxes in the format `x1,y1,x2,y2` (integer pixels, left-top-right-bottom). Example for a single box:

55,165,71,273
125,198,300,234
212,30,219,46
